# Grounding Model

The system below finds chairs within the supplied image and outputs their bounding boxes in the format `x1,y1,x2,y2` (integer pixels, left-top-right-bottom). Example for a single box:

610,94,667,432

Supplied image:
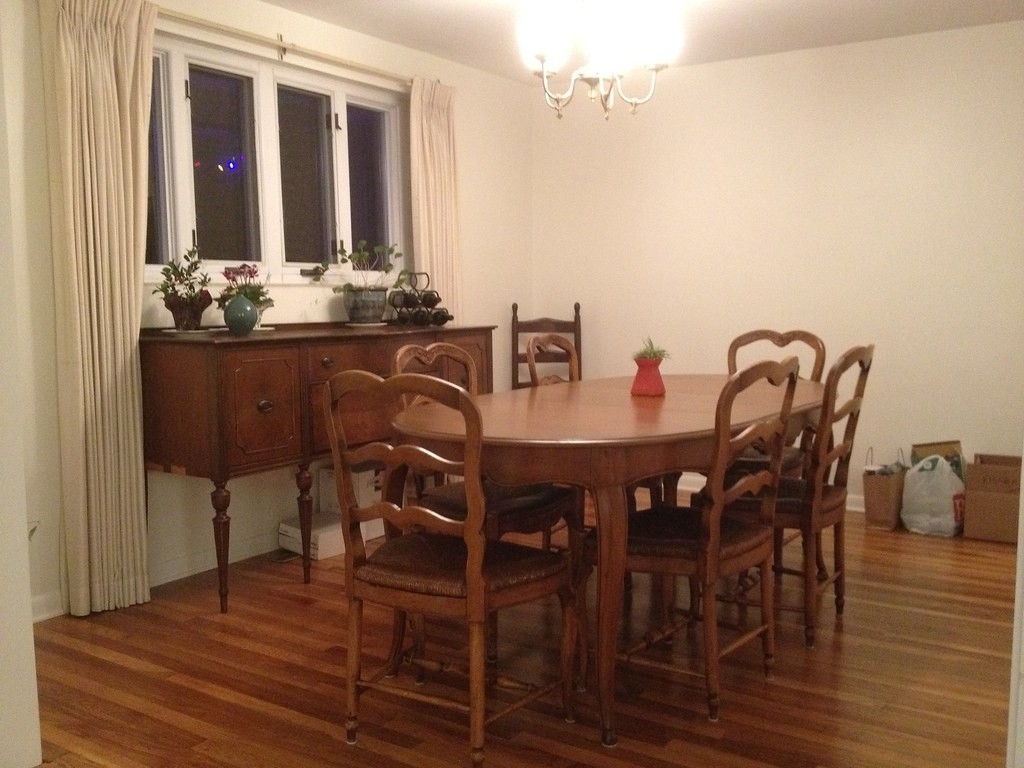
510,300,585,389
527,334,681,620
578,353,800,724
386,342,586,679
689,328,828,582
323,369,584,768
690,342,874,646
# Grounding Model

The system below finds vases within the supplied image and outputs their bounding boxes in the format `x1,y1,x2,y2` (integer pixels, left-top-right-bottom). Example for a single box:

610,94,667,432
163,290,211,332
254,297,274,330
223,291,257,336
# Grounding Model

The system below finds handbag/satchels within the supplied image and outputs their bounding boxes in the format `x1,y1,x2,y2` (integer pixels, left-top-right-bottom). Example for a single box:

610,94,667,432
863,446,910,530
900,456,965,538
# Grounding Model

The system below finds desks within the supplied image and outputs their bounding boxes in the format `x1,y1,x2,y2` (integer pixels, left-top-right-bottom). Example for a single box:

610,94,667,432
135,323,500,614
392,378,834,750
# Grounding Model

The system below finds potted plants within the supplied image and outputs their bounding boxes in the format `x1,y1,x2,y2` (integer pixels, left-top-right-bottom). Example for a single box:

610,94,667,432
630,337,669,398
314,240,412,323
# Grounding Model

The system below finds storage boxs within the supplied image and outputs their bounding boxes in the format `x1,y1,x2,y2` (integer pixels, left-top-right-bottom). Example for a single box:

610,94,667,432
278,458,385,561
911,441,1020,545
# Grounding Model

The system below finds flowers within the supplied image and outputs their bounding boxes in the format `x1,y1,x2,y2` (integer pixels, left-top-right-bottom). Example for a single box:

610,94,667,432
212,262,271,309
153,251,211,301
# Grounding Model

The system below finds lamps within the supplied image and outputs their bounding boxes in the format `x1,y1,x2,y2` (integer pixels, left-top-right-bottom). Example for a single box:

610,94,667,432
512,1,688,119
